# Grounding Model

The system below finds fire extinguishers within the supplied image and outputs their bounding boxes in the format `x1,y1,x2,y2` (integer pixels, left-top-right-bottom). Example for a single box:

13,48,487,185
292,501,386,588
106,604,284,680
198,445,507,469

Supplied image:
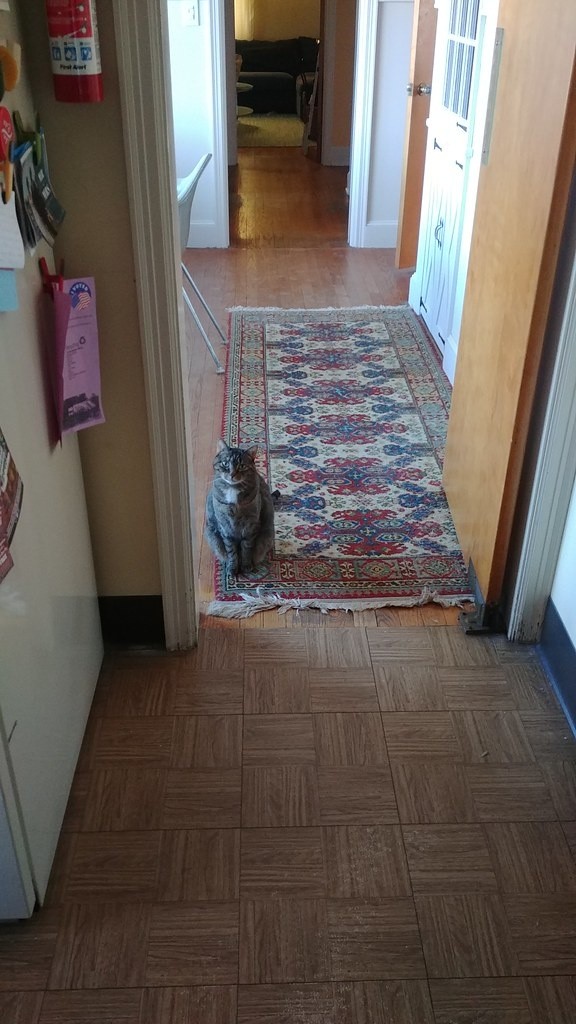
43,1,106,104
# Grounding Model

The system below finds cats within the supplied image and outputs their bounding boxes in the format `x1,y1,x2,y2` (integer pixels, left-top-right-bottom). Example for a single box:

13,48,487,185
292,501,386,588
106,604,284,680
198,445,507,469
206,438,281,577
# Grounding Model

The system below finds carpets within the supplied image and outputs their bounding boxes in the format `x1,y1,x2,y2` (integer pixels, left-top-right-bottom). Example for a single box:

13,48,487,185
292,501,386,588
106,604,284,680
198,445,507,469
207,306,475,619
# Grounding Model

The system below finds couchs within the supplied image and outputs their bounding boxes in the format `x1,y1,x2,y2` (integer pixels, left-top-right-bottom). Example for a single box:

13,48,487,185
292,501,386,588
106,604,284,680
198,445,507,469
235,36,318,114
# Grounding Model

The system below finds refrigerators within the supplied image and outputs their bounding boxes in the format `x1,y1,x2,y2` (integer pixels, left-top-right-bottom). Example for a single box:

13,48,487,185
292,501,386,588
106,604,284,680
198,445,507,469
0,0,105,922
